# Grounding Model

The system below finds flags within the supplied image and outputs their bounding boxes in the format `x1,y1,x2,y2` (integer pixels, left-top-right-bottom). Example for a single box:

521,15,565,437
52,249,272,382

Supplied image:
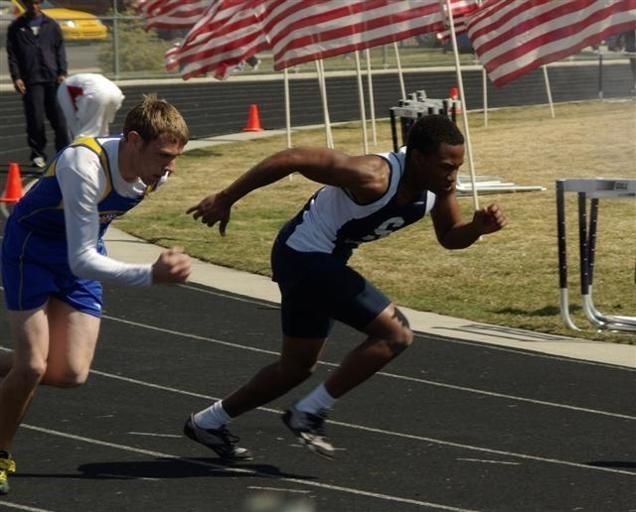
122,0,499,81
462,1,636,86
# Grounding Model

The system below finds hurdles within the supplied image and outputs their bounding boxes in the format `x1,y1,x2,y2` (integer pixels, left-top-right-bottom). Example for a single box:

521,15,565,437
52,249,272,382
556,177,636,334
388,90,546,199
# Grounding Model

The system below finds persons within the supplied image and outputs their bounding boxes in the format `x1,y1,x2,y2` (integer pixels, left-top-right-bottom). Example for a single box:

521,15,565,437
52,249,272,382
57,73,125,138
0,90,193,494
185,113,508,472
7,0,75,168
594,31,636,84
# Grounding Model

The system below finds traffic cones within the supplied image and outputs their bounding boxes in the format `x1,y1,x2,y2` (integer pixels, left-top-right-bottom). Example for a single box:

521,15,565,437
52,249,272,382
448,87,462,112
0,163,24,203
242,104,264,132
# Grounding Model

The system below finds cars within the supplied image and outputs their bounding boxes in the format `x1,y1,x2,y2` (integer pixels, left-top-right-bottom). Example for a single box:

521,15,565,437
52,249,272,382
0,0,108,40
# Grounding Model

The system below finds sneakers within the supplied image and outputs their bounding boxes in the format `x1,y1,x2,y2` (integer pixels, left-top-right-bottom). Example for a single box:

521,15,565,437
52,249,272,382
0,449,17,495
280,398,337,460
182,411,253,461
31,156,47,168
